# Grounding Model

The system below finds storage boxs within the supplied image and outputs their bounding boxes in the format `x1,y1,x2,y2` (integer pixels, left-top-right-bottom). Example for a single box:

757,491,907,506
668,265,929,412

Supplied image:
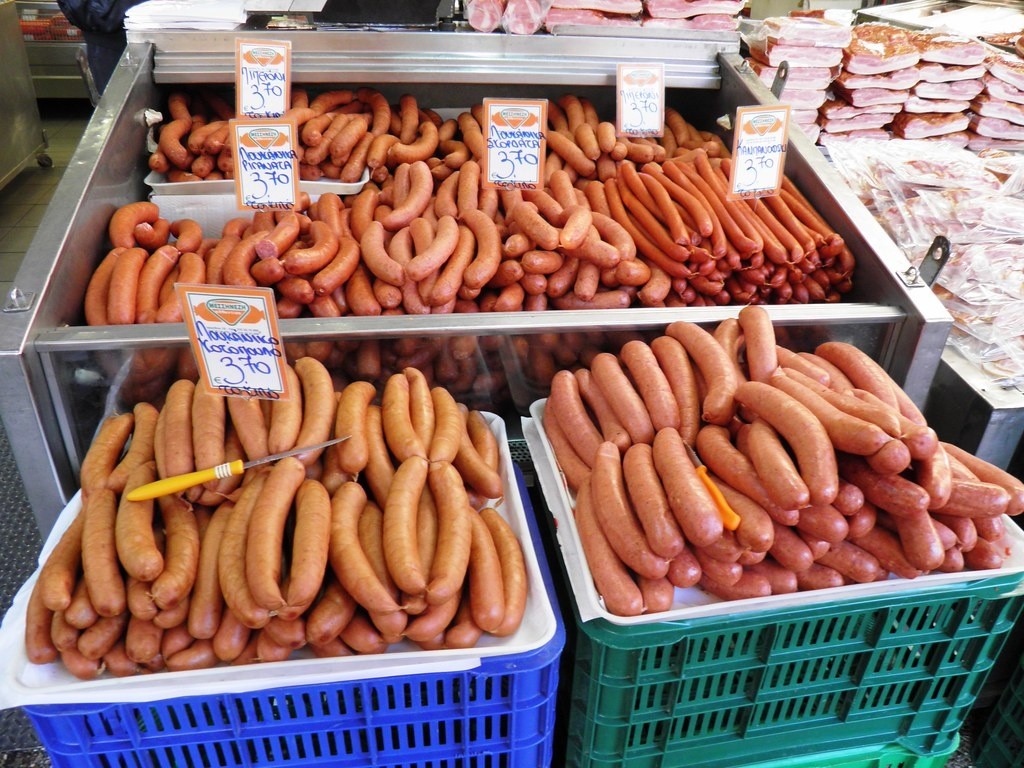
9,413,565,768
529,398,1024,755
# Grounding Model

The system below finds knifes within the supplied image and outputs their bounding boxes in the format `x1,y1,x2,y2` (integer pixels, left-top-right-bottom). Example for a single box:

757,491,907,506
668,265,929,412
682,439,741,534
126,433,354,505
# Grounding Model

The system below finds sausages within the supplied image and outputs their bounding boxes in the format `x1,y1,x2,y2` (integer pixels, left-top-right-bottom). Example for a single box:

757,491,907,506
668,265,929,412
21,90,1024,676
